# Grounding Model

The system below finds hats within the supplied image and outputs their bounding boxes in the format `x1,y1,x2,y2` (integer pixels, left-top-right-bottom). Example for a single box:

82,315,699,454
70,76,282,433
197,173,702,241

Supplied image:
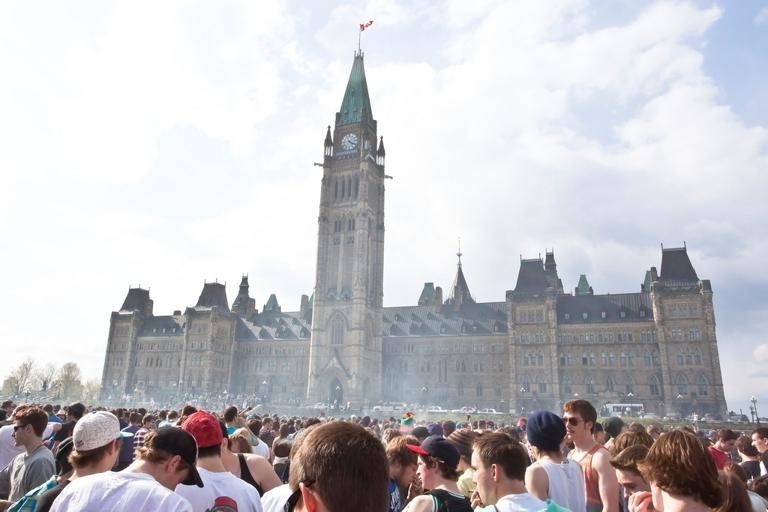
426,405,447,414
452,406,477,414
315,402,336,410
664,412,750,424
477,407,504,416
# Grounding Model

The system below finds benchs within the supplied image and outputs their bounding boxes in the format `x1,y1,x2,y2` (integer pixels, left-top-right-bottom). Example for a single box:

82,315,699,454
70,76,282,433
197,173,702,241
358,20,374,35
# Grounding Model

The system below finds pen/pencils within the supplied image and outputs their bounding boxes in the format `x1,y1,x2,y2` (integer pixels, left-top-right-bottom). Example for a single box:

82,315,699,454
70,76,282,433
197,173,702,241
373,401,409,414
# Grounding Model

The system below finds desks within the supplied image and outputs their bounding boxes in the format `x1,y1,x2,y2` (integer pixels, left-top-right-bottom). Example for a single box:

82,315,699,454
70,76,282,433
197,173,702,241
519,387,527,416
676,394,686,422
99,379,168,403
179,379,184,393
750,395,760,423
335,386,341,409
262,380,267,404
23,387,31,403
627,392,635,417
421,386,427,416
222,389,228,411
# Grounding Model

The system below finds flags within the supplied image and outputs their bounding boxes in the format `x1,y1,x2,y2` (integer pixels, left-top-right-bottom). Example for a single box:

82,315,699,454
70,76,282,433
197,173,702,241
358,20,374,35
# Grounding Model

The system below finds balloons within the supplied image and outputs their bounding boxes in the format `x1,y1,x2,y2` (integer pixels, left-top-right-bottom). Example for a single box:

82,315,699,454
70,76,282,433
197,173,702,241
340,133,358,151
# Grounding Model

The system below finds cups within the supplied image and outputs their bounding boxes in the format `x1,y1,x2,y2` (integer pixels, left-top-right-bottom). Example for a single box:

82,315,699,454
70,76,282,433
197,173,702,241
563,418,585,427
13,425,24,432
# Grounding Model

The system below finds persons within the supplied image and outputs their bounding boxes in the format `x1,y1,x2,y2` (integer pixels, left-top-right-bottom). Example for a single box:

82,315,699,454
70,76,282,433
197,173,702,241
1,400,766,511
561,400,619,512
470,432,563,511
173,411,262,510
524,410,587,511
640,429,723,510
36,410,135,510
283,421,390,511
49,425,203,510
402,435,473,511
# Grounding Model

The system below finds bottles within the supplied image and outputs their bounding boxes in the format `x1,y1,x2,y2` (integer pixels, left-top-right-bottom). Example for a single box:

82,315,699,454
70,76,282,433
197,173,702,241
70,402,85,415
601,418,623,437
72,411,135,452
528,411,567,445
144,425,205,488
182,411,223,449
406,420,478,468
